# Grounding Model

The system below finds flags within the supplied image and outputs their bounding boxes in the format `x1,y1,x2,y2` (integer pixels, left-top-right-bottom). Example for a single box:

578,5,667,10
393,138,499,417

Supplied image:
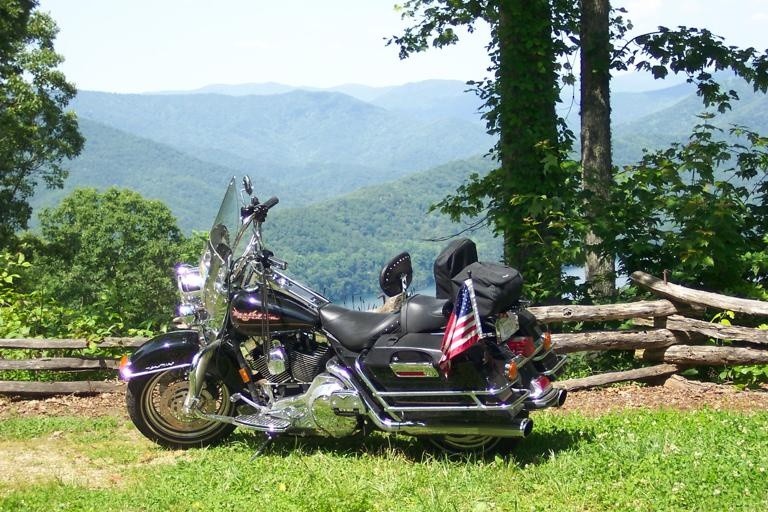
437,280,479,377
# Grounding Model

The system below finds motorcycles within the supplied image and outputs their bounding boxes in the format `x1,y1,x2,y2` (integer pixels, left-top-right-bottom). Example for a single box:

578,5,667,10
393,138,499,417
119,174,568,464
